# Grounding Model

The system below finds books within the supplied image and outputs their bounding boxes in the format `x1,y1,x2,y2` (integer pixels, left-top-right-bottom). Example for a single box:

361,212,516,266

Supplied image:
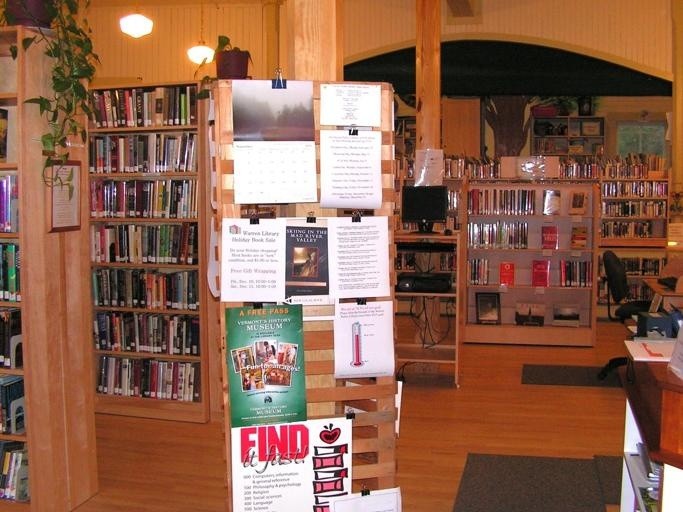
1,108,29,502
624,341,676,362
636,442,661,484
87,84,199,402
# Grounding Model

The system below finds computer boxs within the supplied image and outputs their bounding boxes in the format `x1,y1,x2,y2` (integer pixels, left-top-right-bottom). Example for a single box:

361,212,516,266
395,272,453,292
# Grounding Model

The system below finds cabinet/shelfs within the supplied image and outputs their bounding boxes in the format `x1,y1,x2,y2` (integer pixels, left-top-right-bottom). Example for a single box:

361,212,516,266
87,77,211,425
594,170,670,305
388,96,464,390
1,24,97,512
464,177,599,347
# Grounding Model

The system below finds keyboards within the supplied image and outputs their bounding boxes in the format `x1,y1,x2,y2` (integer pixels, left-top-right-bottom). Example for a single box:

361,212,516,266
398,242,455,251
659,277,679,289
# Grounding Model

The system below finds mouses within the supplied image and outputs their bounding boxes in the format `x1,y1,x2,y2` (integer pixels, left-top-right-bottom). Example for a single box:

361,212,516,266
445,230,452,235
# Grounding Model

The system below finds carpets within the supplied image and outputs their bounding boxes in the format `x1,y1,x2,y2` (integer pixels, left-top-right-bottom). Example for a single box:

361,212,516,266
449,452,623,511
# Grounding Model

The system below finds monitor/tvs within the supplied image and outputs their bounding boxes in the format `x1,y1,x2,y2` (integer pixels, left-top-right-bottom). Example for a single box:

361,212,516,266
402,186,447,234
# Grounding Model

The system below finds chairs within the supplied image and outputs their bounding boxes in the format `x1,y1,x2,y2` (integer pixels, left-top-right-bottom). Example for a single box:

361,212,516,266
602,250,662,339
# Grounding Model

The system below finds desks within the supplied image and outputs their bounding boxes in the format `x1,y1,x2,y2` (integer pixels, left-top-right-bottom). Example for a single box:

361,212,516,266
645,257,683,315
